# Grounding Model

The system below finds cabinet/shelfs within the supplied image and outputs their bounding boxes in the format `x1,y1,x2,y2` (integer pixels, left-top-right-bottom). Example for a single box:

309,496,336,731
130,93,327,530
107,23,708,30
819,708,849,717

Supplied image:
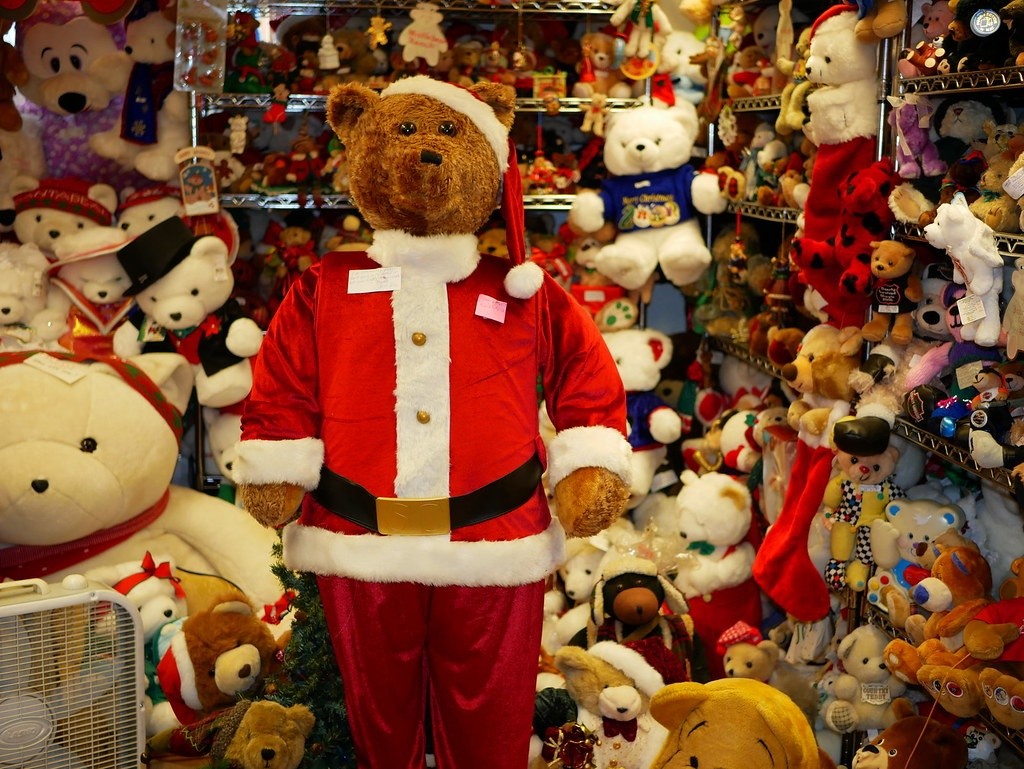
194,0,1024,769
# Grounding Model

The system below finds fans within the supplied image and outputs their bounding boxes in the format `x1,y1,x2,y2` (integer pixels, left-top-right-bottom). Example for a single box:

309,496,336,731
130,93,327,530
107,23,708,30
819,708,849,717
0,574,148,769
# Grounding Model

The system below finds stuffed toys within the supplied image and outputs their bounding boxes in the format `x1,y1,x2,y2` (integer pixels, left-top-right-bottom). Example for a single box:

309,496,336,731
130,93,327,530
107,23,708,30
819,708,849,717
234,77,632,769
1,0,1024,769
